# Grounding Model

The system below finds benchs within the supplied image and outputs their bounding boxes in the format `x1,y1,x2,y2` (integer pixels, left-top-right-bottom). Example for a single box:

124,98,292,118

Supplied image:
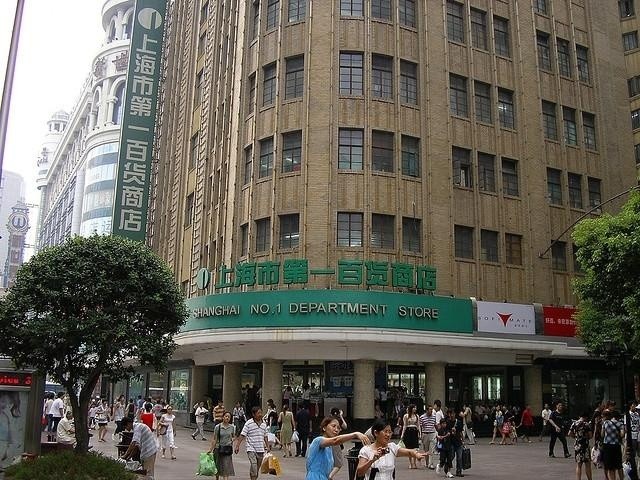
41,441,74,455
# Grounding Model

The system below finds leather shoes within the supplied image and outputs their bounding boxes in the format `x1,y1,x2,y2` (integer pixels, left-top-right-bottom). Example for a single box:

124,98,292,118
565,453,572,458
549,454,555,458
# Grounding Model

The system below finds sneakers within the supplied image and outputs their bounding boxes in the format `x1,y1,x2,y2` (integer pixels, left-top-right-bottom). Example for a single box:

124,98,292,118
436,463,442,476
282,452,305,458
161,454,177,460
445,471,456,479
409,465,436,470
191,435,207,441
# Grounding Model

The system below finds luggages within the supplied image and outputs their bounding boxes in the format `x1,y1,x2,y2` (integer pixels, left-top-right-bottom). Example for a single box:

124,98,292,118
462,445,473,470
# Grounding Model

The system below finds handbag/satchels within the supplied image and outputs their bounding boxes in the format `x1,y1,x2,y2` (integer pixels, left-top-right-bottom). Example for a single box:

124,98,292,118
267,433,276,443
156,414,168,437
620,461,632,479
195,452,219,477
466,421,475,429
260,452,283,477
217,444,234,456
94,405,107,420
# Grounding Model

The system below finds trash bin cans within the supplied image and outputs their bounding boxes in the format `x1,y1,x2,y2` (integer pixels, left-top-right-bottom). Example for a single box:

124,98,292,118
116,432,147,475
344,441,374,480
